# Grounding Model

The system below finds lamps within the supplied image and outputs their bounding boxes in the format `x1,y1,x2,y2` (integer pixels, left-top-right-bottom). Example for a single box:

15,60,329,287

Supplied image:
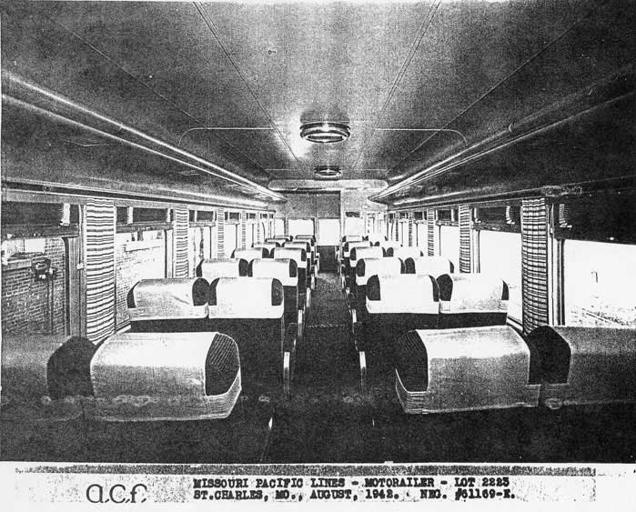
312,166,339,177
301,123,350,144
